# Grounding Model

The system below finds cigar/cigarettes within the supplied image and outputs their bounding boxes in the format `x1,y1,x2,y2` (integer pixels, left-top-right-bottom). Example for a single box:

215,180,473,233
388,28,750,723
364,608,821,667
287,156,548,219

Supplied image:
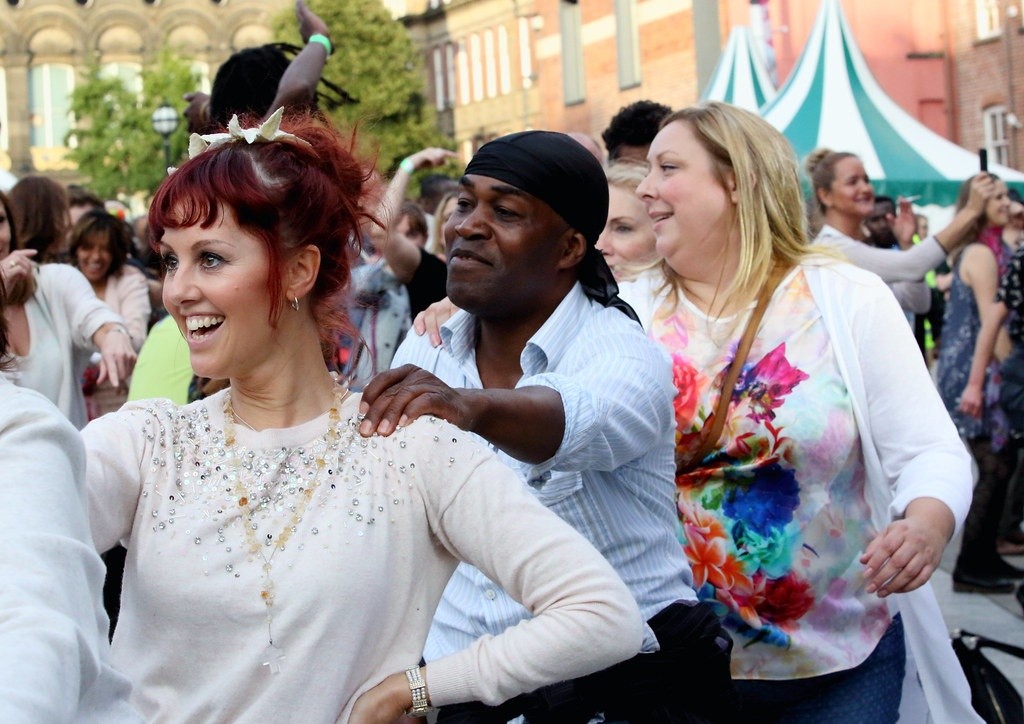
896,195,921,204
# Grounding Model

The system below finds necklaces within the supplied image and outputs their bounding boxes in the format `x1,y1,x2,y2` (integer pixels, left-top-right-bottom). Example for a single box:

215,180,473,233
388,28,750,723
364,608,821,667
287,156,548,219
220,370,341,676
232,387,349,431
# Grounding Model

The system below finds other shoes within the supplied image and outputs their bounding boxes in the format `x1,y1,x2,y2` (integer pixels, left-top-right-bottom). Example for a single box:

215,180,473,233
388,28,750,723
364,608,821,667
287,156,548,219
952,552,1024,592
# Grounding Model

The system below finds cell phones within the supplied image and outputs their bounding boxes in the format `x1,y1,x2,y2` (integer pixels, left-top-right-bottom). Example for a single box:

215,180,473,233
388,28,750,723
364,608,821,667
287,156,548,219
979,148,987,171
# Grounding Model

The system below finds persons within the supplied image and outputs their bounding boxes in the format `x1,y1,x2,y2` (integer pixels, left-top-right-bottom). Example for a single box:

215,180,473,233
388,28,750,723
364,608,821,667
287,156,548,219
76,112,642,724
0,265,146,723
0,0,1024,724
356,128,734,723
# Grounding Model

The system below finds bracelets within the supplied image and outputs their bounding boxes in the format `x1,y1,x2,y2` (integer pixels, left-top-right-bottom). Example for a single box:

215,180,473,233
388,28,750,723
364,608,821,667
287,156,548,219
400,160,415,176
404,664,433,718
109,328,130,334
932,234,949,255
197,100,211,123
309,35,333,57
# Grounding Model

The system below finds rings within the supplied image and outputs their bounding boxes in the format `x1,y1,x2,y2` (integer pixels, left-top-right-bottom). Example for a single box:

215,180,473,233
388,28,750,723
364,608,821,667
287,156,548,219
9,260,16,267
963,405,968,412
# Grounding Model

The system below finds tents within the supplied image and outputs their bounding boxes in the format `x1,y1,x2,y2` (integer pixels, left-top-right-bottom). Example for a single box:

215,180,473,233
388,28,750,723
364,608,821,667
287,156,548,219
756,0,1024,209
695,23,774,114
